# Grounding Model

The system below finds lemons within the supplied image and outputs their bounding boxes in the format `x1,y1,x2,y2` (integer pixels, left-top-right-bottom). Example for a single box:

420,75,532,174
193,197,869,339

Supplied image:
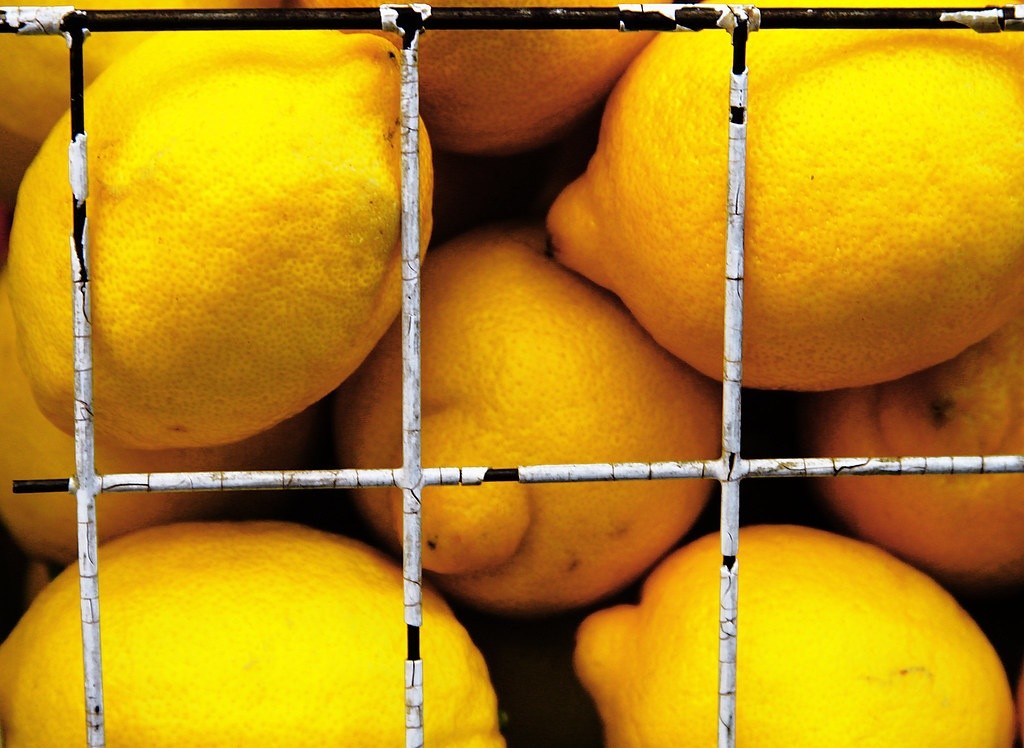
0,0,1024,748
